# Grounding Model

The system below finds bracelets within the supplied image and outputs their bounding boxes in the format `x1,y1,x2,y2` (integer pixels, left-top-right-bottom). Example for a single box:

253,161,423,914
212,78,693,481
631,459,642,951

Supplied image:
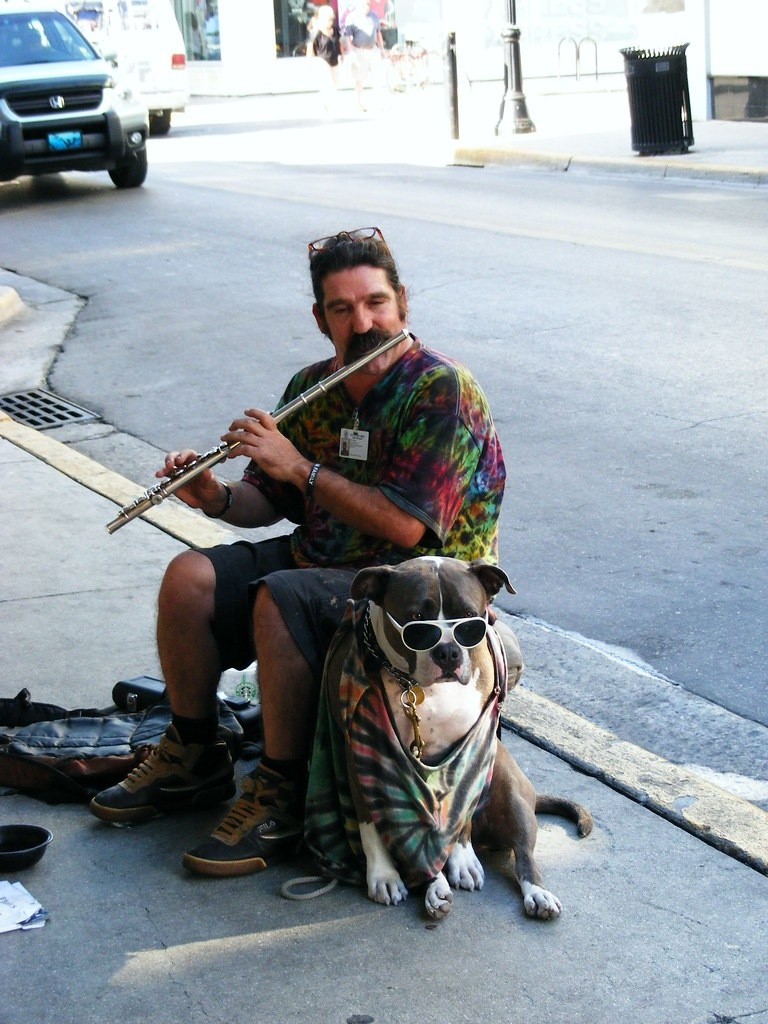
202,481,232,519
305,463,321,496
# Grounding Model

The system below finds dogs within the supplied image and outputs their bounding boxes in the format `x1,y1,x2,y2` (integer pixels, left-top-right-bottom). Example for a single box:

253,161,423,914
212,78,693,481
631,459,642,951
325,552,592,922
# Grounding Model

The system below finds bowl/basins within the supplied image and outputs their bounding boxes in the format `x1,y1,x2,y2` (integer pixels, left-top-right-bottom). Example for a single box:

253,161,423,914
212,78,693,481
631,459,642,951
0,823,54,872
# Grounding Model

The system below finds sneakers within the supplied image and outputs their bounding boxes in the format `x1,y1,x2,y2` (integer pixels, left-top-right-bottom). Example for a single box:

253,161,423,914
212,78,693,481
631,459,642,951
89,723,237,823
184,762,307,877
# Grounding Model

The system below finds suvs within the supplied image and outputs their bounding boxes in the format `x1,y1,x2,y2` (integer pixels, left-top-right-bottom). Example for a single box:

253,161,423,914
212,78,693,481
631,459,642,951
0,3,149,188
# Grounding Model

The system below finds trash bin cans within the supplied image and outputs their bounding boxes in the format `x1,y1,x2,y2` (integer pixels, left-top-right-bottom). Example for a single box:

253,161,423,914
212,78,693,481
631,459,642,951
620,44,693,158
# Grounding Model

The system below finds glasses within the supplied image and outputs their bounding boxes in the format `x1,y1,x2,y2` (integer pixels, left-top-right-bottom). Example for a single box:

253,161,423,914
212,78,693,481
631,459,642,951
384,610,490,652
308,227,386,259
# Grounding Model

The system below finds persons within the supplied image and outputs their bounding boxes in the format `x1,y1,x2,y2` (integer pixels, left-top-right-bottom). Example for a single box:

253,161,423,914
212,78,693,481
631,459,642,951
17,29,52,59
91,226,506,873
341,442,348,455
345,0,386,111
309,5,342,103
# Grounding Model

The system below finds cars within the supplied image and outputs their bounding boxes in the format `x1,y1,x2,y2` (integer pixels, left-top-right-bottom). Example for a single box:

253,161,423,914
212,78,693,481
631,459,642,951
61,1,190,136
